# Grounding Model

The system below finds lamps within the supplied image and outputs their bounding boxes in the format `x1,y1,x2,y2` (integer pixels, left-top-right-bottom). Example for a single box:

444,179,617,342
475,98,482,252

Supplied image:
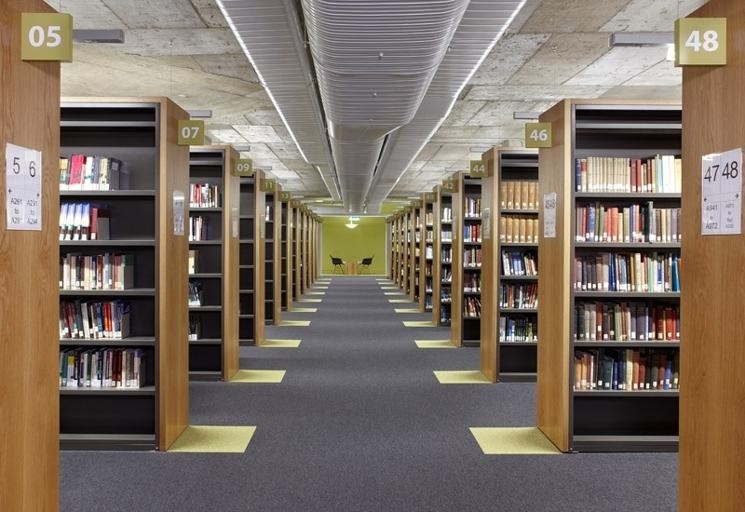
344,216,359,229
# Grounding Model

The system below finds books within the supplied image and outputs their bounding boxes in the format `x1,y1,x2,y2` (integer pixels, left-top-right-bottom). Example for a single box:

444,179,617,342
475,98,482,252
502,249,537,276
190,182,220,208
407,219,411,229
463,246,482,267
463,271,481,292
441,203,452,221
440,304,451,321
426,278,433,291
426,262,433,276
574,154,683,194
408,246,410,255
59,152,122,192
500,213,538,245
265,206,273,220
499,316,537,344
188,281,204,305
427,230,433,241
573,250,680,293
415,261,419,269
60,348,146,389
465,296,480,318
59,200,112,241
425,294,433,309
188,250,201,273
426,212,434,224
441,265,452,283
574,348,679,390
463,223,482,243
408,257,410,267
442,224,452,241
59,251,135,291
441,243,452,263
499,280,538,311
415,246,420,256
189,216,209,241
415,274,419,285
576,201,681,244
573,299,681,341
415,231,421,242
440,286,451,302
465,197,480,218
501,179,539,211
417,217,420,227
188,314,201,341
408,233,410,242
59,298,129,341
426,244,433,259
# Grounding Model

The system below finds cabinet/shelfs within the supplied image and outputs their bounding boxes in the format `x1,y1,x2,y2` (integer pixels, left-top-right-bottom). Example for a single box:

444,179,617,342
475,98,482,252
57,97,192,453
189,143,241,384
241,167,267,348
410,201,419,306
301,205,308,294
1,0,63,512
481,145,539,428
418,192,433,319
281,192,293,312
264,180,283,327
292,198,301,302
404,207,411,294
536,97,679,456
675,3,742,512
389,212,404,289
308,209,320,289
451,169,481,370
433,183,451,340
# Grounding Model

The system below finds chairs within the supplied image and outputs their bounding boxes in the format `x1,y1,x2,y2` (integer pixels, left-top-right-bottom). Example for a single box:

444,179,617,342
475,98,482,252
329,254,345,274
360,255,375,275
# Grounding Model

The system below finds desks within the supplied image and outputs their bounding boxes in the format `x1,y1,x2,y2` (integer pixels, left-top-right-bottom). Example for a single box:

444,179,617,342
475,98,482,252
345,257,360,275
